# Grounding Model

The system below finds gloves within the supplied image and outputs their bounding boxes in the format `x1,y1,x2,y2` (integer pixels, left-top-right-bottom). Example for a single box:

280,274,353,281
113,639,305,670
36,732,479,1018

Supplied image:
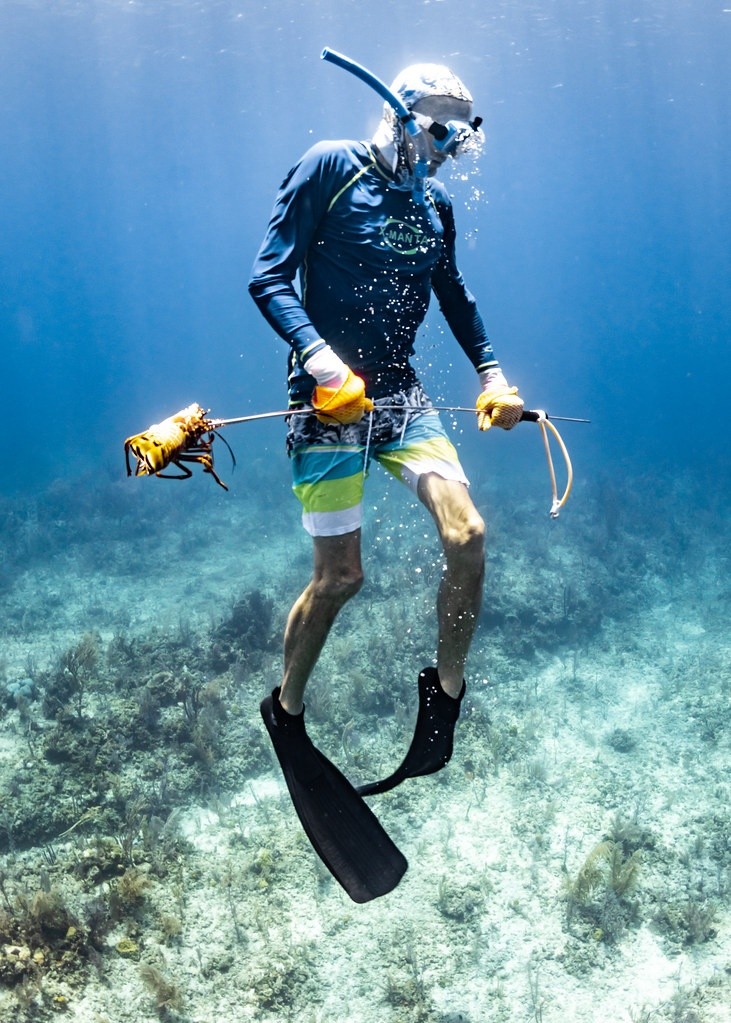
303,344,375,428
476,368,524,432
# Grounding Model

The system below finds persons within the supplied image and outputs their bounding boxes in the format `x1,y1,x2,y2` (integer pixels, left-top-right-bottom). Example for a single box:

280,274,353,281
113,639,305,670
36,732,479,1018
247,62,526,786
